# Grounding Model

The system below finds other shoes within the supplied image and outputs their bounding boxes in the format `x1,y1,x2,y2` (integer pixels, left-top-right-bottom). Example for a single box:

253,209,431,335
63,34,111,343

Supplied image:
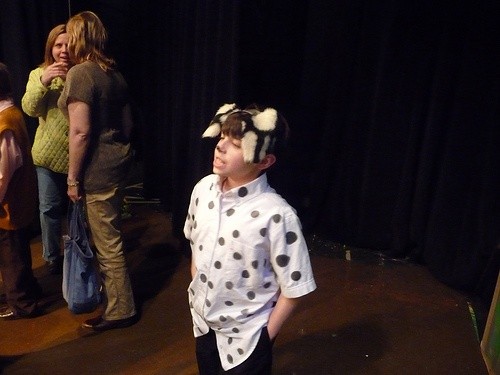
82,313,141,332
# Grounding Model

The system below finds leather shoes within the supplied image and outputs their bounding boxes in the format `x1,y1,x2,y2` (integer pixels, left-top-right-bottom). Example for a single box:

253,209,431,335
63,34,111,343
0,305,17,319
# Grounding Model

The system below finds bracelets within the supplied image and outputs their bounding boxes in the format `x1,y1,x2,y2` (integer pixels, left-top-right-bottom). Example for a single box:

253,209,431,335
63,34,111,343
67,177,80,187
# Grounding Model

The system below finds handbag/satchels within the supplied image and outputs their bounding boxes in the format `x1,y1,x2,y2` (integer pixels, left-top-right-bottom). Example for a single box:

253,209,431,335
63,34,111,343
60,199,105,315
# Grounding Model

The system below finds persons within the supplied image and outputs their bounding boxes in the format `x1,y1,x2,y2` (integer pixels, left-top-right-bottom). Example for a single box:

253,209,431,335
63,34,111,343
183,103,317,375
20,23,76,285
0,63,43,319
57,11,139,334
